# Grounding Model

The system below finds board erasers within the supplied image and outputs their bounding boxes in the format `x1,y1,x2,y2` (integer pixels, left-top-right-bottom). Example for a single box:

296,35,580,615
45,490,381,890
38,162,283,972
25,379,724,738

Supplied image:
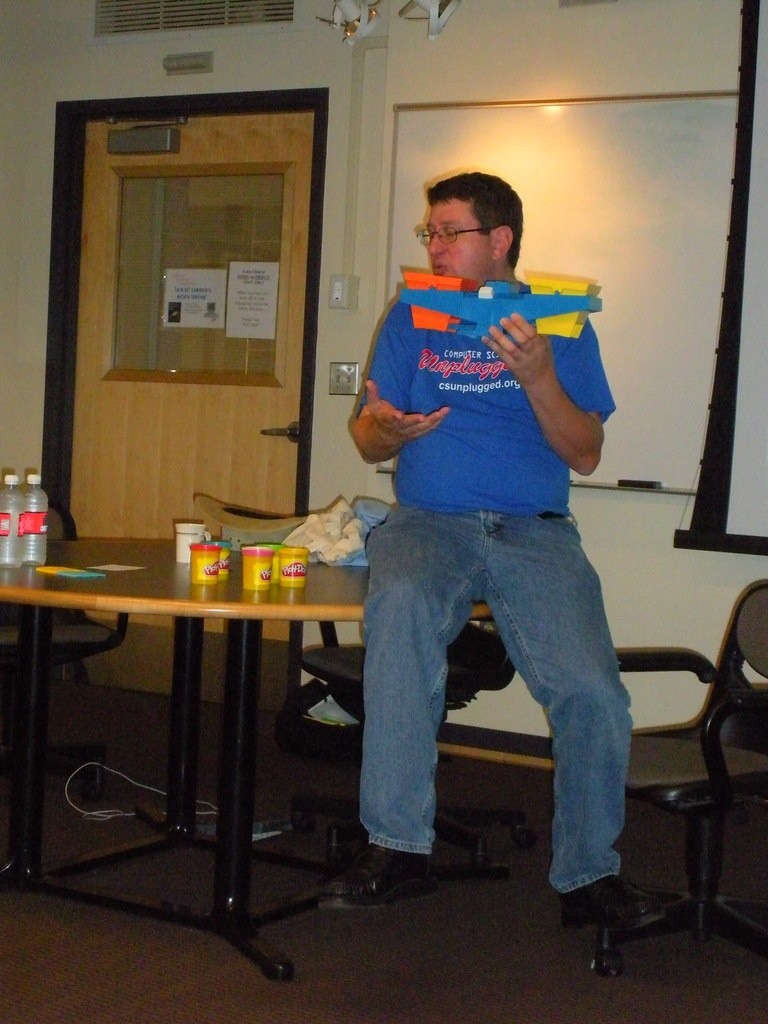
617,479,664,490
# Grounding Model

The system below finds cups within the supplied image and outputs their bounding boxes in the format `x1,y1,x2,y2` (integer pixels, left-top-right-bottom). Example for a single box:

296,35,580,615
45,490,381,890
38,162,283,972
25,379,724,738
241,543,308,590
189,541,232,584
175,523,211,563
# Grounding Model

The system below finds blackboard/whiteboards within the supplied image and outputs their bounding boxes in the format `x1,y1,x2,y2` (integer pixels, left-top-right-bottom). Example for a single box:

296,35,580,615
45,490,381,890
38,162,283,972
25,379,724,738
373,87,737,496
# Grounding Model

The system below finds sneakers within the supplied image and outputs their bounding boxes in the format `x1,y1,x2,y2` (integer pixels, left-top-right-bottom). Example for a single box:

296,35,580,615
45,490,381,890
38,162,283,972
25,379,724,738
317,843,438,904
558,874,667,932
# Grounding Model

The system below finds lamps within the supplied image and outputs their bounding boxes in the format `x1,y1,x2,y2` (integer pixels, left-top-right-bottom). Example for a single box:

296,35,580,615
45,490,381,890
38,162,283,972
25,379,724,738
398,0,460,39
332,0,384,47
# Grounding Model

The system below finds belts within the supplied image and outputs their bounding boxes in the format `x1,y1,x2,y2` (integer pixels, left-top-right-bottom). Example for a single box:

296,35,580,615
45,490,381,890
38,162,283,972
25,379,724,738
537,511,565,520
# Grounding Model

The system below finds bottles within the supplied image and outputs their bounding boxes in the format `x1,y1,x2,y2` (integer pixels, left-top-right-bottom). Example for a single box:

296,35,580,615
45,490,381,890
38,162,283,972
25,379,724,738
0,475,22,568
21,474,48,567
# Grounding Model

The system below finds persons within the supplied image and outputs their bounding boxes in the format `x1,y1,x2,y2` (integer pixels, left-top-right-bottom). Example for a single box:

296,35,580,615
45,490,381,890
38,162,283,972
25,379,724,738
324,170,666,929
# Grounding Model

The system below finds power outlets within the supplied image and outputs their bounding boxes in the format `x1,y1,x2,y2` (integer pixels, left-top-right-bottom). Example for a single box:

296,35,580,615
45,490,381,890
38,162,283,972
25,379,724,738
329,362,357,396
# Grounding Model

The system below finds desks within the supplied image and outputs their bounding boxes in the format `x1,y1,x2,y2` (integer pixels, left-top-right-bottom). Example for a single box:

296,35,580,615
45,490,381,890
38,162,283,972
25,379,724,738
0,536,494,981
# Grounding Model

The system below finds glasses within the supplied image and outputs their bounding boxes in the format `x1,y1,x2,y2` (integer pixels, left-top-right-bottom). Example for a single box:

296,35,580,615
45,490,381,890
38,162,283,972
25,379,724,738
415,227,491,246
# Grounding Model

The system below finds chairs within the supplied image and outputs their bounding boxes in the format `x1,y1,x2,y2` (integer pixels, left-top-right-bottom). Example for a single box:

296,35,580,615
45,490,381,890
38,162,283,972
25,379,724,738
590,579,767,977
0,499,128,802
271,615,541,875
